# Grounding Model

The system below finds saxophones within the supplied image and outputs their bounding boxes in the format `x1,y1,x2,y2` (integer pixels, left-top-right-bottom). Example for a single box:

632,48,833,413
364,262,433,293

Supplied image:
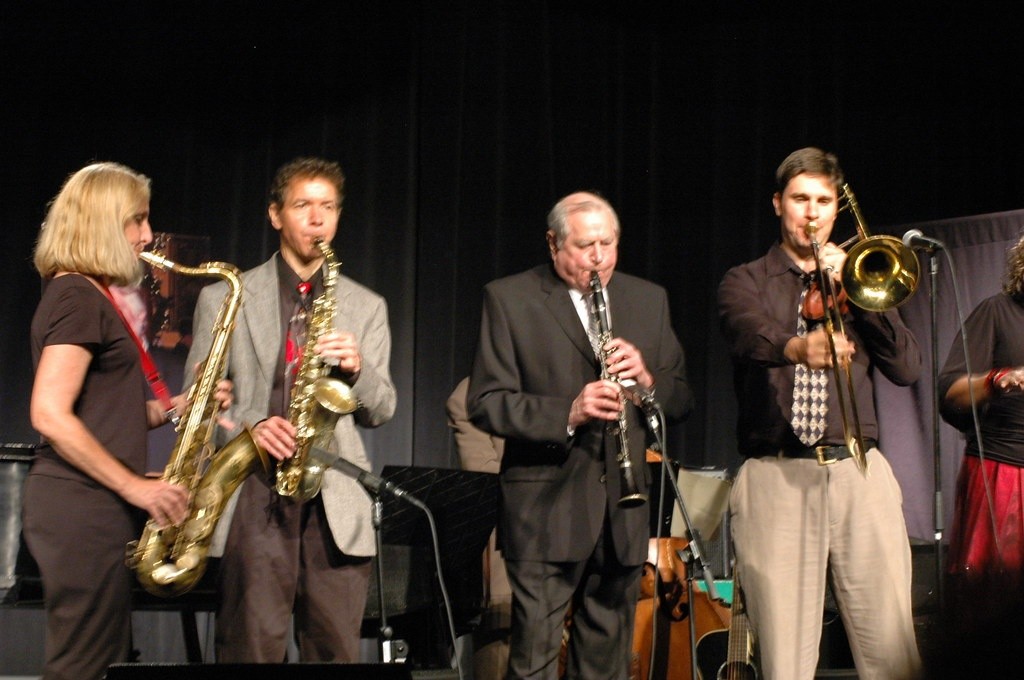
273,233,362,506
103,251,274,603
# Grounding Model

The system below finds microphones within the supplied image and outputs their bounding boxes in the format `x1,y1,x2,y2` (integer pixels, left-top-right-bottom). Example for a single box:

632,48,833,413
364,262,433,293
331,457,404,497
902,229,946,251
620,378,661,410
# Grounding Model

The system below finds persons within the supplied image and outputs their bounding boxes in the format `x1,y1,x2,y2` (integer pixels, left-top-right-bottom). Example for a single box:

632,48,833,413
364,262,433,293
938,238,1024,576
720,148,923,680
914,563,1024,680
22,163,234,680
446,193,684,680
181,159,398,662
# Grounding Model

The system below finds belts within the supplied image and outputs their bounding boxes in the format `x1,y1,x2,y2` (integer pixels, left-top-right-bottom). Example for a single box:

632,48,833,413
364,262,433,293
754,437,875,465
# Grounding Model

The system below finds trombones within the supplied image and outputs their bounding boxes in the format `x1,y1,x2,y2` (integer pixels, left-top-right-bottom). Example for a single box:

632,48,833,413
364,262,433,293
803,180,924,478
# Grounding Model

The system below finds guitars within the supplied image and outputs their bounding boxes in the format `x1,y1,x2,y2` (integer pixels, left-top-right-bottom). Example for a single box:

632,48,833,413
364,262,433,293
692,554,765,680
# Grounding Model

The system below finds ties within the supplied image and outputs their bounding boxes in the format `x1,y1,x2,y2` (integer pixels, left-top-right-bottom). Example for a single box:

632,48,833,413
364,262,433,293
583,293,604,356
790,273,828,448
282,281,314,419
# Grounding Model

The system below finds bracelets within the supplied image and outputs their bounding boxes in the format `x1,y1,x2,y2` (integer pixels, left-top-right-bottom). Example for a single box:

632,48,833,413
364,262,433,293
986,368,1011,393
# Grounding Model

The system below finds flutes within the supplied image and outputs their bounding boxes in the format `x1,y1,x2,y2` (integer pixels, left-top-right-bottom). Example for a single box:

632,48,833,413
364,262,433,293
590,268,649,510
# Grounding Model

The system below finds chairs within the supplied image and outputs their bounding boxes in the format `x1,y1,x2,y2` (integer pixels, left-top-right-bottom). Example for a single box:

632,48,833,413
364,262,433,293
127,580,218,667
